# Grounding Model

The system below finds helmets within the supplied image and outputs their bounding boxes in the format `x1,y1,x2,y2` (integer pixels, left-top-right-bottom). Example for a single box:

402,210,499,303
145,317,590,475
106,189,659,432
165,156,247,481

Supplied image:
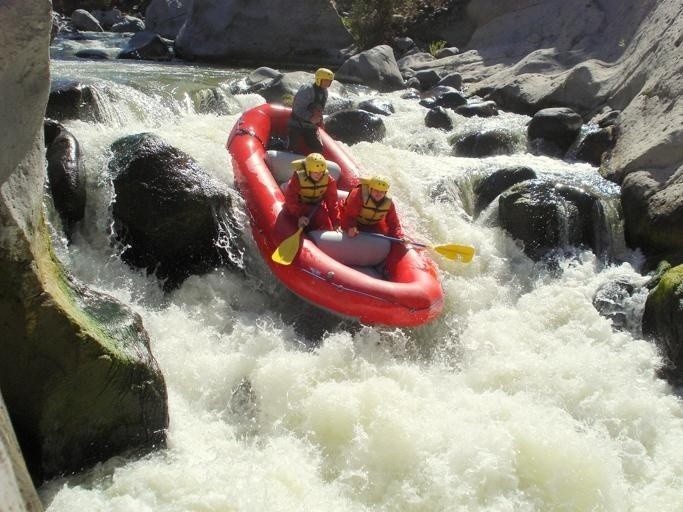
315,67,334,86
304,152,327,177
368,175,390,195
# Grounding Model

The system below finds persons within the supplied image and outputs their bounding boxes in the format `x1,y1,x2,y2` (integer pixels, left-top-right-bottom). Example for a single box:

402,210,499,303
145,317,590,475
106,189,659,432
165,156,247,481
284,68,412,244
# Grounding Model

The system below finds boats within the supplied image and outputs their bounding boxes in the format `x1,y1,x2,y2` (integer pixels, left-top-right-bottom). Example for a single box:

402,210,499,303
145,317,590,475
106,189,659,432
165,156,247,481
227,102,445,330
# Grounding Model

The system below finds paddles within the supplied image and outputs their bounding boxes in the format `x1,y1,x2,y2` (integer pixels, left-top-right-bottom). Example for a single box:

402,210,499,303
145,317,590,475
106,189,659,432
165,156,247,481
272,204,322,265
359,232,474,263
